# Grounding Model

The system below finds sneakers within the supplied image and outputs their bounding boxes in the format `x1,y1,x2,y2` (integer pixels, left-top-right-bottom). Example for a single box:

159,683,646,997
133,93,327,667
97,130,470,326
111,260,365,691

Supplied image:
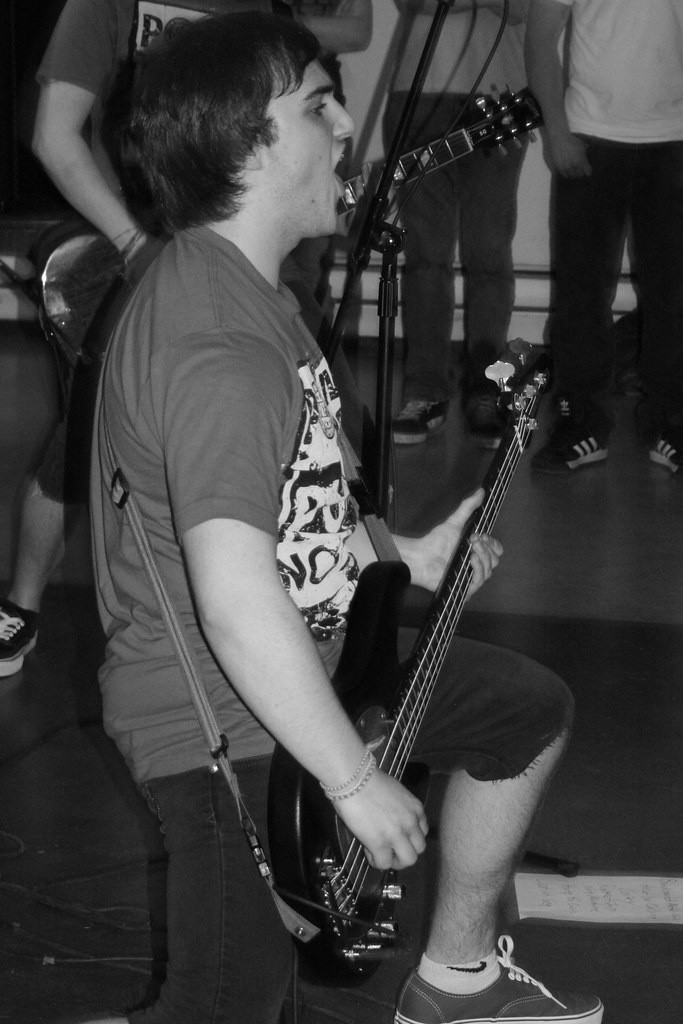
392,399,448,444
647,436,683,481
391,934,606,1024
461,395,509,450
530,392,611,475
0,599,40,678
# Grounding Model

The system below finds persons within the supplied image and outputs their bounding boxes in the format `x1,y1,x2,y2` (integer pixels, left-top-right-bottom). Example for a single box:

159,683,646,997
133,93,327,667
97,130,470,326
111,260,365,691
87,11,610,1022
1,0,683,679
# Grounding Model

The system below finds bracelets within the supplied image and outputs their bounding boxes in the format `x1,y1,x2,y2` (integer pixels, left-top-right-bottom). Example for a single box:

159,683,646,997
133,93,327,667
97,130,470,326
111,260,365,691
319,748,376,801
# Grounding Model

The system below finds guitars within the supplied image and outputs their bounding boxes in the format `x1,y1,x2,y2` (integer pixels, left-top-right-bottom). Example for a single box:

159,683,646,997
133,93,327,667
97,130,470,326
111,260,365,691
264,337,558,991
39,86,547,372
